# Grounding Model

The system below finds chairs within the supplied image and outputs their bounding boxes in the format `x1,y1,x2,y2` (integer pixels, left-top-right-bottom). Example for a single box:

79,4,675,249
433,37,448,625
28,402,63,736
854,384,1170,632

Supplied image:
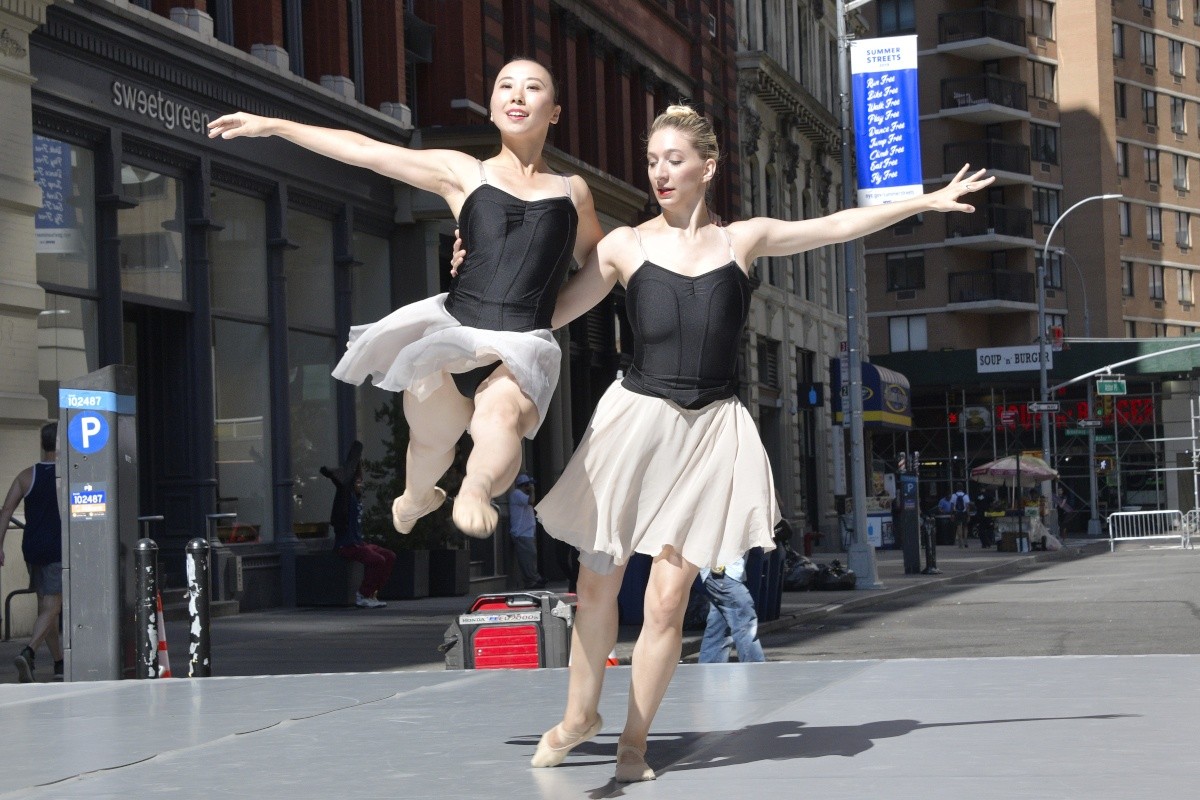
954,92,989,108
840,516,854,552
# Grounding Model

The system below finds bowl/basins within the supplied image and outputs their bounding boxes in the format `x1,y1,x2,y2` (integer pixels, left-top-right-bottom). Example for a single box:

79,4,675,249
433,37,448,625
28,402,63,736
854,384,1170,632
293,521,330,539
215,525,260,543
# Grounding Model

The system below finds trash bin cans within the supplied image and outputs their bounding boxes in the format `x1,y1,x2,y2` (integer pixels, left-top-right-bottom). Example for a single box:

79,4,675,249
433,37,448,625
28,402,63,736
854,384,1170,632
438,585,578,671
573,519,790,632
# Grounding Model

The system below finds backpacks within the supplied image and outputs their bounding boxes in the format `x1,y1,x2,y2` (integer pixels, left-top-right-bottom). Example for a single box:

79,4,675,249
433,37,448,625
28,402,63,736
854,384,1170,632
955,493,965,510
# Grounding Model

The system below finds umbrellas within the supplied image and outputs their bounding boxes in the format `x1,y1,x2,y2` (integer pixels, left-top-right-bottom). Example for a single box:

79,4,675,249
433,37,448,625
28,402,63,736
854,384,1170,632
971,454,1058,509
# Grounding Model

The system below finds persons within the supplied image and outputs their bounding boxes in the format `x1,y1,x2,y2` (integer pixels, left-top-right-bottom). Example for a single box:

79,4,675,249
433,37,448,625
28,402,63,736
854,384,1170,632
334,480,396,609
207,59,603,541
450,104,996,781
698,551,767,662
0,421,65,683
509,474,548,589
939,482,1067,549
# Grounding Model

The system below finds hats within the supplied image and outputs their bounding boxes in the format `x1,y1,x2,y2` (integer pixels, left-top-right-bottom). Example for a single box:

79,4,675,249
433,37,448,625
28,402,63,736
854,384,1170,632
516,473,534,485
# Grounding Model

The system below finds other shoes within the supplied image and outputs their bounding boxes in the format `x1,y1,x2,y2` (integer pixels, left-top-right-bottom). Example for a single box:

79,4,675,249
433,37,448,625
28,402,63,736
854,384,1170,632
53,659,64,680
14,646,36,683
356,590,387,608
524,583,545,589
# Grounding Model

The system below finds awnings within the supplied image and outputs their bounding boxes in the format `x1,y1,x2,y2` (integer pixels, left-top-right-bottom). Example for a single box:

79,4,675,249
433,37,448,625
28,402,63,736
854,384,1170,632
829,357,911,431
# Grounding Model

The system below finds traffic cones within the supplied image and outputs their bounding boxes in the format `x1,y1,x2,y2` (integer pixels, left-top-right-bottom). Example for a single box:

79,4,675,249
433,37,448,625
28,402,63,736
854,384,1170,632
156,596,174,680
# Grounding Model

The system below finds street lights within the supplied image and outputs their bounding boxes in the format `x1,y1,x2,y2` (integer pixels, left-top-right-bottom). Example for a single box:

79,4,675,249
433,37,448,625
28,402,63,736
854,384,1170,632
1054,247,1102,535
1038,191,1126,540
